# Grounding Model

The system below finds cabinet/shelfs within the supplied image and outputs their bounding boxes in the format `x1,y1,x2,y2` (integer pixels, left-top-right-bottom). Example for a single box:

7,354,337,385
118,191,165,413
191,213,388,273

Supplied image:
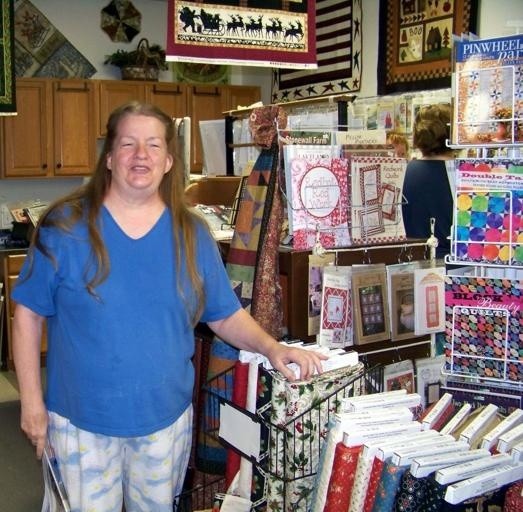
0,77,261,181
439,33,522,401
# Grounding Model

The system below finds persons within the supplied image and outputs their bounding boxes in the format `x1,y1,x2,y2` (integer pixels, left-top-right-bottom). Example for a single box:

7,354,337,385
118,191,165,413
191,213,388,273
398,103,461,259
11,101,329,512
387,134,409,158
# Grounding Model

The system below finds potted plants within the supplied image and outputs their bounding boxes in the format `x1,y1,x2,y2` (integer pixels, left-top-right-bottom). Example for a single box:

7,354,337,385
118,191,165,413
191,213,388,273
102,38,167,81
